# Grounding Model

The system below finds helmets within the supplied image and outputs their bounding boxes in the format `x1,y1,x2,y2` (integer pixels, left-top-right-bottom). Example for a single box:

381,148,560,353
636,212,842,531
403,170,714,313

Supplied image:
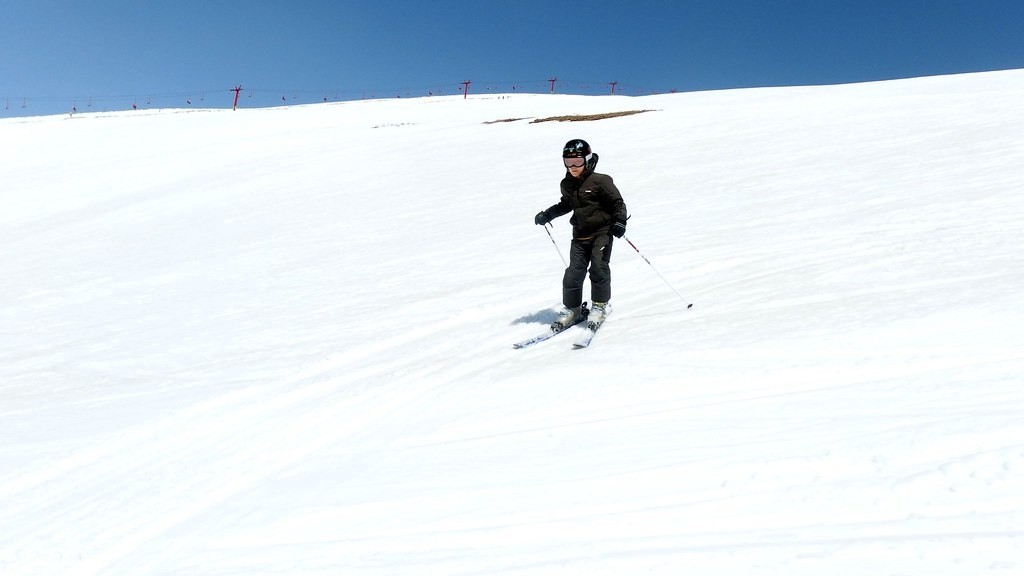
563,139,592,158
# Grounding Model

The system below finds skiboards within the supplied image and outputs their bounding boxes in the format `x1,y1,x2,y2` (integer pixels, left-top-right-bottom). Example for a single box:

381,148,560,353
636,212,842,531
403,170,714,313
512,301,613,348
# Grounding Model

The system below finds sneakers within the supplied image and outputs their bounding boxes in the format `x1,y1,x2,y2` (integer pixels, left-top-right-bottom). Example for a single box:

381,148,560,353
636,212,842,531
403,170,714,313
551,308,582,329
587,302,608,325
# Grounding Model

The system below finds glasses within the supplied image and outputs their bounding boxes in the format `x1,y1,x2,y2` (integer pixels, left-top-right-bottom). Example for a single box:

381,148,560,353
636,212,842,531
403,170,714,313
563,158,586,168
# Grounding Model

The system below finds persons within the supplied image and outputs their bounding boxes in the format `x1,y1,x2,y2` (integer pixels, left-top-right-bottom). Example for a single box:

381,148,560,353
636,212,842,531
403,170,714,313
535,140,627,331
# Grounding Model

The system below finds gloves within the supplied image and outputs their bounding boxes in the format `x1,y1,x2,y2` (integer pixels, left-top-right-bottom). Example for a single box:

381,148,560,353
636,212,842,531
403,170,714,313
612,217,626,238
535,211,549,225
